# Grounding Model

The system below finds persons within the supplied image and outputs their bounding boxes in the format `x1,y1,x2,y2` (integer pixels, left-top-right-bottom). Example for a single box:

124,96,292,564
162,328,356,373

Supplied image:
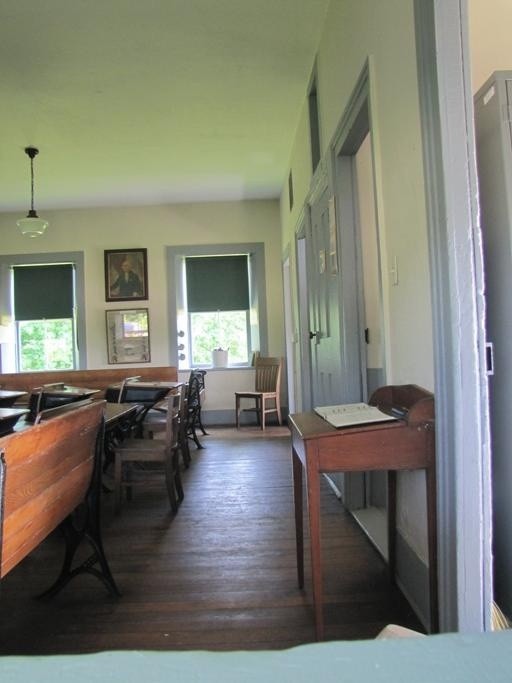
109,260,143,297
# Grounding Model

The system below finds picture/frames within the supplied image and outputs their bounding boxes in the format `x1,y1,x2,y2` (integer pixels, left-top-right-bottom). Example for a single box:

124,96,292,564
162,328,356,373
105,308,151,365
104,249,148,302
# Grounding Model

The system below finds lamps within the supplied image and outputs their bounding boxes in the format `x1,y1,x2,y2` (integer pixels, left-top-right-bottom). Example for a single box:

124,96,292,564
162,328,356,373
16,148,48,237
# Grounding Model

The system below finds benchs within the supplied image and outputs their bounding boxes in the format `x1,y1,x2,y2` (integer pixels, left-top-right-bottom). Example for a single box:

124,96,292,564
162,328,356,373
0,401,120,605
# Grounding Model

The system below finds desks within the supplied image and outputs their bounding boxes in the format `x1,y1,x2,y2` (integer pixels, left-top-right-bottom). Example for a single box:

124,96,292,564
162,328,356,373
288,383,436,642
0,390,30,437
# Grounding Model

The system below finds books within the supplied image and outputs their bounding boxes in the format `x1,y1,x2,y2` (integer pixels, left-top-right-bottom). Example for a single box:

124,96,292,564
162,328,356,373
311,401,398,429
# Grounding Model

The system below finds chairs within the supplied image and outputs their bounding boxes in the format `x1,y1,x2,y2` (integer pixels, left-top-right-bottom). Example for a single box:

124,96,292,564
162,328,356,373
33,369,206,510
234,353,283,432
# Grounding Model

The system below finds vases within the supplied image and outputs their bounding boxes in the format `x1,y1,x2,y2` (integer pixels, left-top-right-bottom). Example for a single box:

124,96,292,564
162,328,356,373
213,350,228,368
